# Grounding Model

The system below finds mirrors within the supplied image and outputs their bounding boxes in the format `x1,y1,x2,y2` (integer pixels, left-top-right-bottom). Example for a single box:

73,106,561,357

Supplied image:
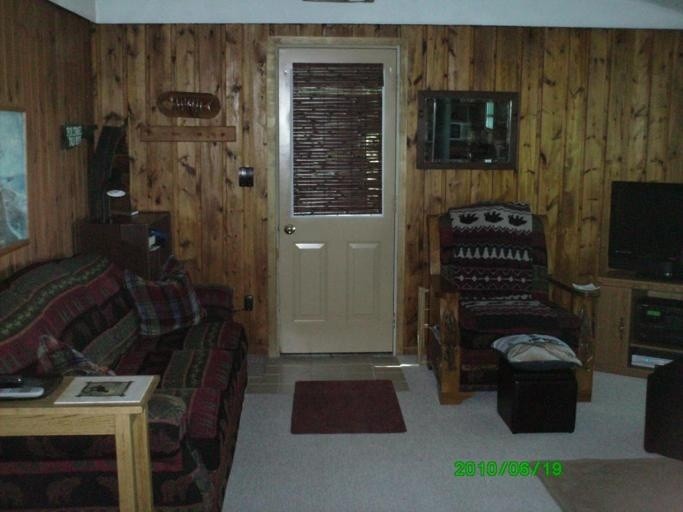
415,89,521,172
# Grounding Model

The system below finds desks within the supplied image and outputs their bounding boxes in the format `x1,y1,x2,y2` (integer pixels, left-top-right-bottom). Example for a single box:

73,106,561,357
1,375,159,510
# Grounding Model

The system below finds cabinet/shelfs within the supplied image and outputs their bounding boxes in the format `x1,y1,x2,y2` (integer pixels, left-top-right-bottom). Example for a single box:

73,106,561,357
598,273,682,376
74,210,174,281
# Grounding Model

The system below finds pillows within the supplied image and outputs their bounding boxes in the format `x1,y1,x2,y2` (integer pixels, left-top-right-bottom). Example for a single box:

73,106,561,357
490,333,582,369
34,335,117,379
123,261,209,337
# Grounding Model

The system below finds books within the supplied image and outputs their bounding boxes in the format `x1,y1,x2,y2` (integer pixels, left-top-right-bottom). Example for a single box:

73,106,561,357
53,376,153,404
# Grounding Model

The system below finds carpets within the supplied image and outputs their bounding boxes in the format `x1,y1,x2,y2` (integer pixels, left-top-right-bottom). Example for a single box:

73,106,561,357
291,379,407,434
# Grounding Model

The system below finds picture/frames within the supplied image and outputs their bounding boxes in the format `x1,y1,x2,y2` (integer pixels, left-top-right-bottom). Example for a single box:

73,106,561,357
0,108,31,254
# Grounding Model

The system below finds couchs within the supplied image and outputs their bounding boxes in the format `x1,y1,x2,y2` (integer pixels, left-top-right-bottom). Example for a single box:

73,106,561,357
426,204,600,408
1,256,248,511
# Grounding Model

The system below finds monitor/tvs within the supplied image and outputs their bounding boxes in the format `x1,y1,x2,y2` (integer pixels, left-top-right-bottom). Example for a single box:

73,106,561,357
607,180,683,283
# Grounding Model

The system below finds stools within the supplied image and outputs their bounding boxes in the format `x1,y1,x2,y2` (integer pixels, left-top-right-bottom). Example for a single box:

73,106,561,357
497,371,578,434
643,361,683,462
